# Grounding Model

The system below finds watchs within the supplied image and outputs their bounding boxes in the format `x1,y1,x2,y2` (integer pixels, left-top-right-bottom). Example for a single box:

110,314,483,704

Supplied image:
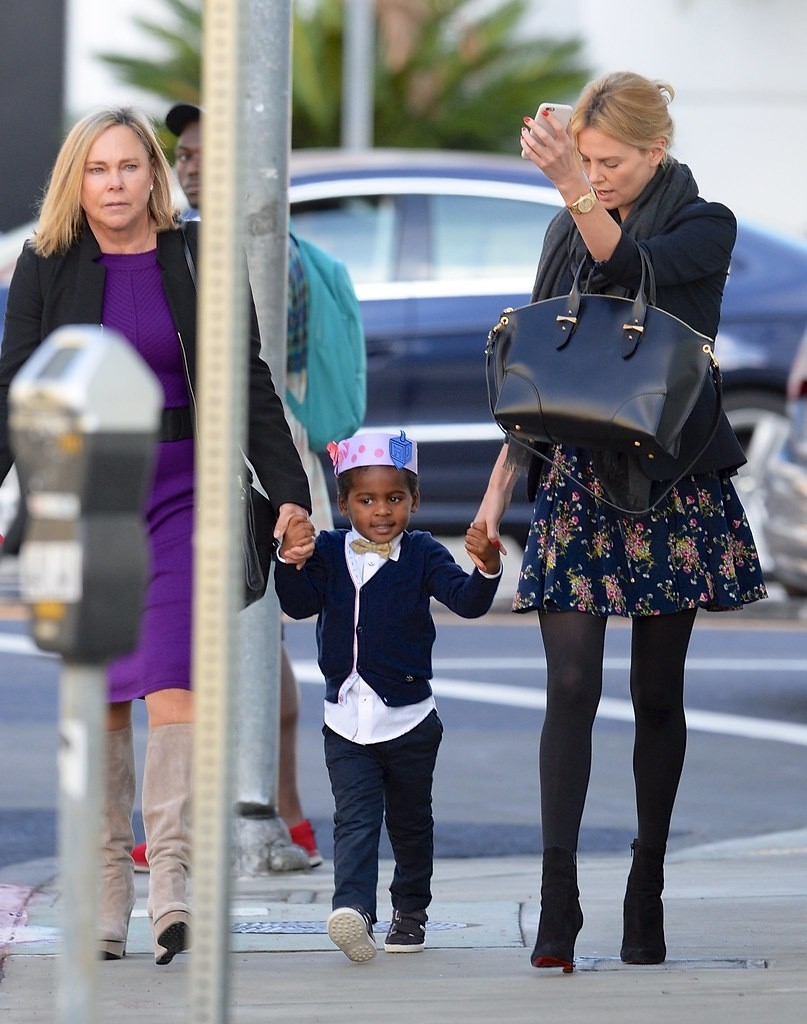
565,186,596,215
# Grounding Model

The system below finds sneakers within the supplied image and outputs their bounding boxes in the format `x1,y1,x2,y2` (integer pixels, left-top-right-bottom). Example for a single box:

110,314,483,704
326,904,378,963
383,907,428,953
129,841,152,874
284,820,324,866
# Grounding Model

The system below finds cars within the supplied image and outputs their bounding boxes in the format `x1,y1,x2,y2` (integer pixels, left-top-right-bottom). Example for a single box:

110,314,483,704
1,145,807,539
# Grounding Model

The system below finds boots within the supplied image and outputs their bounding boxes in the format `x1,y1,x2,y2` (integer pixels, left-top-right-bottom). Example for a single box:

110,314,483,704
142,722,197,967
531,842,584,972
94,727,136,959
620,838,667,965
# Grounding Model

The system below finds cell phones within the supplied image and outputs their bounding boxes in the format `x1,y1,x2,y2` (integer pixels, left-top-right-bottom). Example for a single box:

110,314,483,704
522,102,572,158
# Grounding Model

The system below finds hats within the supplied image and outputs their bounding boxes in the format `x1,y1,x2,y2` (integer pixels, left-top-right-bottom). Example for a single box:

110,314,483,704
327,431,419,474
165,102,202,138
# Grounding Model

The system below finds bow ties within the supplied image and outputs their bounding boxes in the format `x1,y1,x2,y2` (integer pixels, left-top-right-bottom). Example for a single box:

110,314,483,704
349,539,394,561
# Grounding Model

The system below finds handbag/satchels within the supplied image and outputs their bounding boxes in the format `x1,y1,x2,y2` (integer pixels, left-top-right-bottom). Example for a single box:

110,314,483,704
485,231,724,515
239,484,270,614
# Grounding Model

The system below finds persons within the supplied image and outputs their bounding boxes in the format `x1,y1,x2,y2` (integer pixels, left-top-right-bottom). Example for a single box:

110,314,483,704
131,104,335,873
275,432,503,962
467,72,771,974
0,110,315,965
